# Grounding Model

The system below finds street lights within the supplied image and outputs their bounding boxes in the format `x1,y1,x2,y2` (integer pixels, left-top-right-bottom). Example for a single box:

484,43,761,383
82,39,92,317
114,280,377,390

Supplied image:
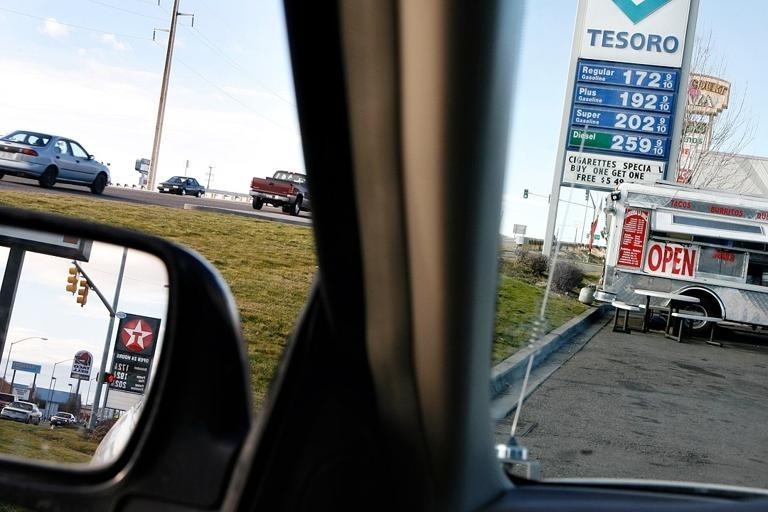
0,335,99,416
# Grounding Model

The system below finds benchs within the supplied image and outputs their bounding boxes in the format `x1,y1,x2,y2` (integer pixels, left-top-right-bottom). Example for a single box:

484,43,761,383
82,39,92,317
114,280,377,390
612,300,723,347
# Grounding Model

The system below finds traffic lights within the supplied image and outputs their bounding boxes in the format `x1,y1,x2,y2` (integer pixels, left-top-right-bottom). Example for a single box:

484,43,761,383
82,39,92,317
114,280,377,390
65,264,90,309
584,189,591,201
523,189,529,200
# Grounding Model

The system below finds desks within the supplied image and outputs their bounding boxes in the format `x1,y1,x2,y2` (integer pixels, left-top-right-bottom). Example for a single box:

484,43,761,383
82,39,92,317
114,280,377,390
634,289,700,336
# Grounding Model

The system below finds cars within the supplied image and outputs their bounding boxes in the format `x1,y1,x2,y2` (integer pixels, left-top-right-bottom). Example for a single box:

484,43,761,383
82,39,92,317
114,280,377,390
0,129,112,196
2,400,43,425
157,176,206,198
50,411,78,427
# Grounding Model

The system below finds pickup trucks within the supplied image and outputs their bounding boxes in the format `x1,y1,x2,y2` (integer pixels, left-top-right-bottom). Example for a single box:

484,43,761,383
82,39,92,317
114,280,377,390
249,168,312,216
